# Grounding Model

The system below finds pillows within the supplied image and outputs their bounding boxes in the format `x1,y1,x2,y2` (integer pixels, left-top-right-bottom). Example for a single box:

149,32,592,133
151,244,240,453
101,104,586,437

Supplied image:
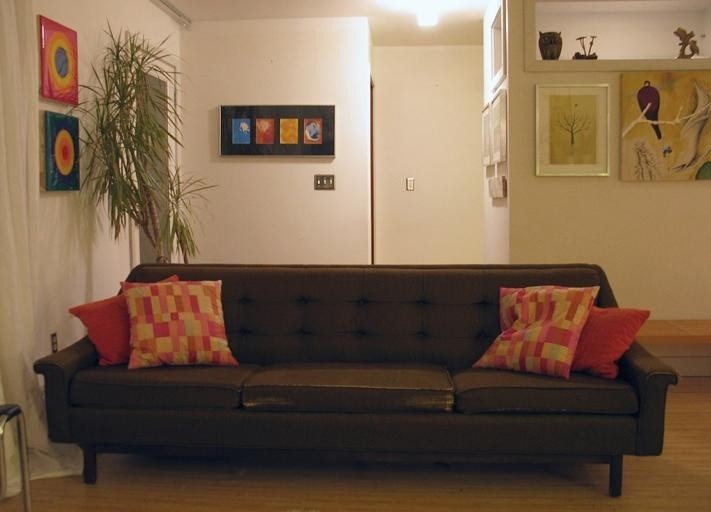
473,286,651,382
67,274,240,371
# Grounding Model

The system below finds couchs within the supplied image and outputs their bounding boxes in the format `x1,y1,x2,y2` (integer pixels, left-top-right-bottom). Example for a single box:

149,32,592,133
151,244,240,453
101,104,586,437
33,263,679,498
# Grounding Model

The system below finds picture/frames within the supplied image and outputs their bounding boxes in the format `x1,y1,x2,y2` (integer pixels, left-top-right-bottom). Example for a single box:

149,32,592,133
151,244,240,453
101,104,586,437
480,1,508,168
219,103,337,159
535,83,612,178
